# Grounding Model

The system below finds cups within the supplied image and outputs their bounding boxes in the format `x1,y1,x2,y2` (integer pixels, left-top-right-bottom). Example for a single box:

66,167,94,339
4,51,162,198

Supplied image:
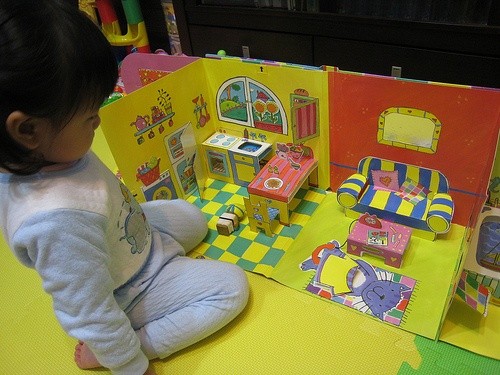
254,0,494,26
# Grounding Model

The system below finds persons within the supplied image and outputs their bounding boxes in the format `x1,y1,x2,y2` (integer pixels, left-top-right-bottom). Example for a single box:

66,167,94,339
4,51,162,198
0,0,250,375
